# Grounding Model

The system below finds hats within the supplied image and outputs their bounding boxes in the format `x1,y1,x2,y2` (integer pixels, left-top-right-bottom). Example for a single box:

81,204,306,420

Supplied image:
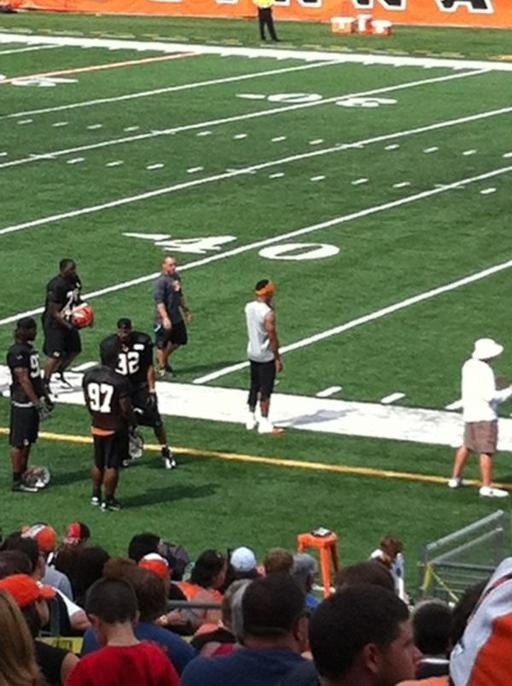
229,545,256,570
471,338,504,362
21,521,56,552
63,520,90,546
140,552,169,580
0,572,56,609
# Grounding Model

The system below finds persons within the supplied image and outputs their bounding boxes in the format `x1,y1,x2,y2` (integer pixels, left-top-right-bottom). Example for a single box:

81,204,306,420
99,318,177,471
253,0,279,43
243,280,284,434
82,337,136,512
447,338,511,499
0,521,511,685
41,259,94,398
7,318,46,494
153,256,192,378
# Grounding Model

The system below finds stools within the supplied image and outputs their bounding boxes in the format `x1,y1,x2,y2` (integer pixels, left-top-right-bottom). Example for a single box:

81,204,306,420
295,533,342,601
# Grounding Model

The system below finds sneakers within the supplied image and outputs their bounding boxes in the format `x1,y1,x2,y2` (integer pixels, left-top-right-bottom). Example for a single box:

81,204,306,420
162,450,177,469
21,472,46,488
11,481,39,494
52,370,72,391
101,498,121,512
256,418,284,434
448,475,462,489
90,495,101,506
478,485,510,497
246,413,257,430
157,368,176,381
